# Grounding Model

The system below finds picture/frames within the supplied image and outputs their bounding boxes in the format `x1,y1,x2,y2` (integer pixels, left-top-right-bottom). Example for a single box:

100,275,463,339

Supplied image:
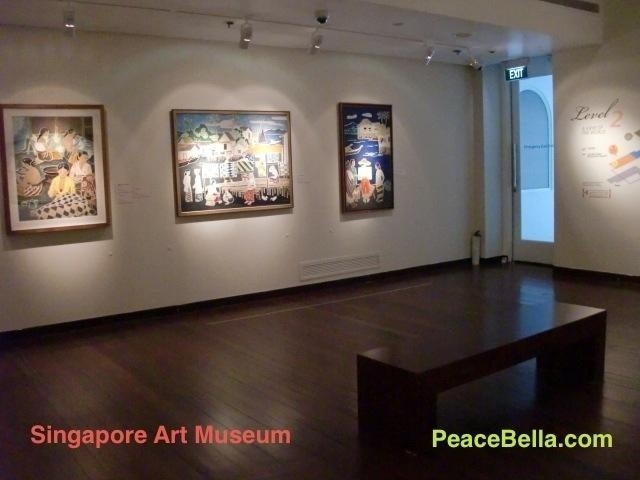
338,101,393,214
0,102,112,236
171,107,293,217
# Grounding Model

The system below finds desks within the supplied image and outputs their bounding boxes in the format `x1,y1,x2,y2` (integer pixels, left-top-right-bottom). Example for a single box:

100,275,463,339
356,303,608,454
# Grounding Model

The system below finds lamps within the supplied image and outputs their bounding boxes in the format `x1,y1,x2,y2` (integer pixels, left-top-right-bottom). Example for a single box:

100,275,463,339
241,16,253,42
312,27,323,48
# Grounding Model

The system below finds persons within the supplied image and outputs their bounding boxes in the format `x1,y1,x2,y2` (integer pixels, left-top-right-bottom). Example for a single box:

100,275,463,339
19,125,93,200
345,157,385,206
181,164,279,207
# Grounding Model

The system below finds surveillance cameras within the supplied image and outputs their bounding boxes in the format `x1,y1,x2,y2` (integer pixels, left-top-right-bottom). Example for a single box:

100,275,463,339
315,9,329,24
